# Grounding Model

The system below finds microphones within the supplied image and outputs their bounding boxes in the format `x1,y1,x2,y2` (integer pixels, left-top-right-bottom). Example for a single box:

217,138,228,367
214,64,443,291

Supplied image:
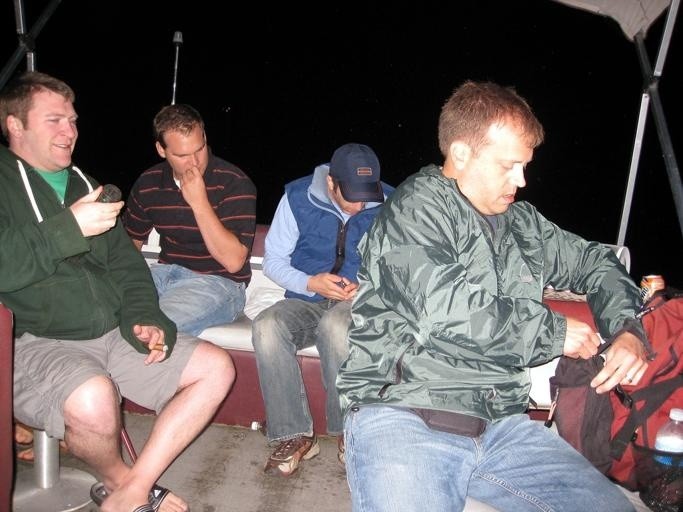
67,183,124,264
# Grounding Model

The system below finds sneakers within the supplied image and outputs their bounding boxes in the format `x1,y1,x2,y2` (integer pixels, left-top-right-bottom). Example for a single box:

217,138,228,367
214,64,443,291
263,434,320,478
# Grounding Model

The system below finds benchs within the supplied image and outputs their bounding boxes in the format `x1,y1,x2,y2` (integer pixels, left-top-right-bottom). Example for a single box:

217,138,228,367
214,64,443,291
116,225,633,439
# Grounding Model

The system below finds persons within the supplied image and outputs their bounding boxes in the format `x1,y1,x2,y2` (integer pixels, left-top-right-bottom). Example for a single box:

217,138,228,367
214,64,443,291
15,106,259,466
335,83,658,512
251,145,399,480
0,71,237,512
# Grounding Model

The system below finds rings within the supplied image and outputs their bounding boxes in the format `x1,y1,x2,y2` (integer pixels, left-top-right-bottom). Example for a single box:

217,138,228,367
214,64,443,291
624,375,634,384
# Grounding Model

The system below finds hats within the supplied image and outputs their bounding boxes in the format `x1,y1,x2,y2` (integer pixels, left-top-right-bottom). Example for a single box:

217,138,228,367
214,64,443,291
328,142,385,204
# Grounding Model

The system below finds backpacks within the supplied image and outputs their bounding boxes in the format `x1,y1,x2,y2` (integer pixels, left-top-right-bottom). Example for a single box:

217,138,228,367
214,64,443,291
549,285,683,512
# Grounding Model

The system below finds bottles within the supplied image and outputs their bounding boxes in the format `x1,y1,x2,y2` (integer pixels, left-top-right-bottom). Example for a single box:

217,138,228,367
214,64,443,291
652,407,683,479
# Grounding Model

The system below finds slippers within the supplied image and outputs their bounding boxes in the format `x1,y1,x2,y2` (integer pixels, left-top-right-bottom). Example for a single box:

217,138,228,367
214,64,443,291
89,478,190,512
